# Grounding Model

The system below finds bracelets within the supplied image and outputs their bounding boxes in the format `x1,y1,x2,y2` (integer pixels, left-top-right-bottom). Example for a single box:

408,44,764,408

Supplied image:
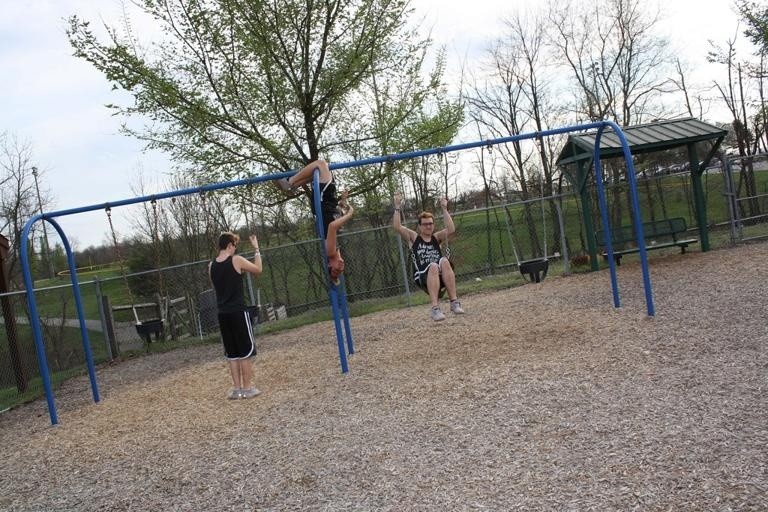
254,252,260,256
394,208,400,214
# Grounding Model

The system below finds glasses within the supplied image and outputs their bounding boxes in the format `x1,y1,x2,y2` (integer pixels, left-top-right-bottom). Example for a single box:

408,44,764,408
421,222,433,225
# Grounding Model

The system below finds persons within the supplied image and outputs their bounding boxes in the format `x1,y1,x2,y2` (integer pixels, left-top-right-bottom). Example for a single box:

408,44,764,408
275,159,354,286
392,193,465,322
208,231,263,400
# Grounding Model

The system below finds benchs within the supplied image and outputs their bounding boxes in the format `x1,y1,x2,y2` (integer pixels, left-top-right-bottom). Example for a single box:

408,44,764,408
594,217,698,265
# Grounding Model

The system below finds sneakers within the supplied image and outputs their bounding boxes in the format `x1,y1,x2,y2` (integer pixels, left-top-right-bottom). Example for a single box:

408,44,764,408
272,179,296,197
242,389,261,399
449,301,463,314
226,389,242,400
430,308,445,322
277,177,292,192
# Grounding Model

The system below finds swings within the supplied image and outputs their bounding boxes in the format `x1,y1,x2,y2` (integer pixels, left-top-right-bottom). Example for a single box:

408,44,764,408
388,152,451,298
199,184,262,327
488,137,549,283
106,200,165,343
